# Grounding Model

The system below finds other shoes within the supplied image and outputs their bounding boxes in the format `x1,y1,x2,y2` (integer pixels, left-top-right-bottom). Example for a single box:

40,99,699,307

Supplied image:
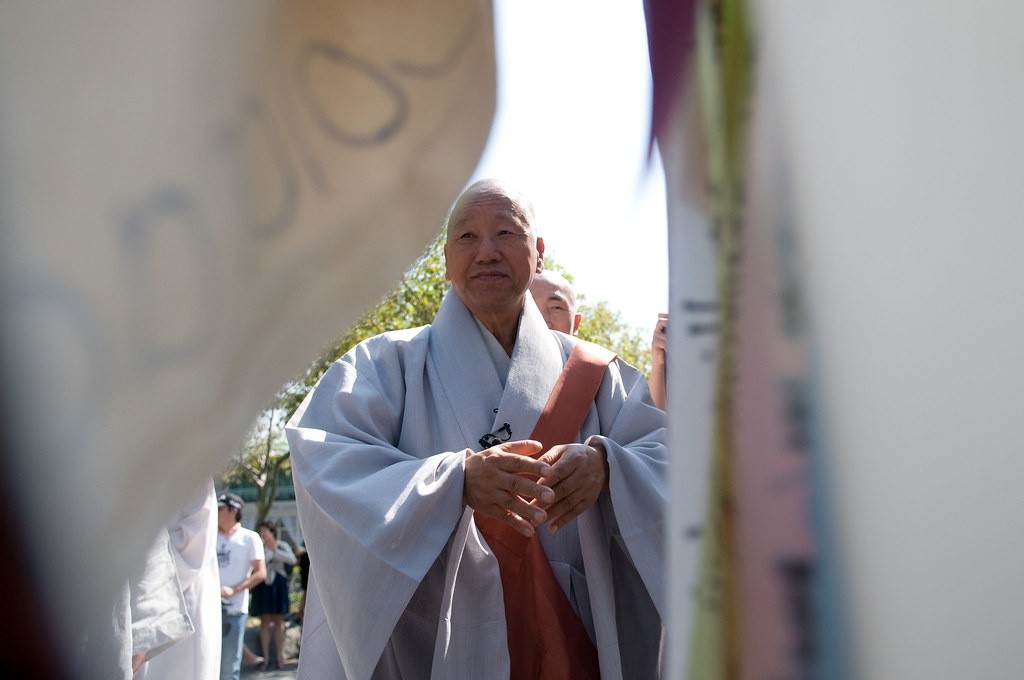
242,656,265,665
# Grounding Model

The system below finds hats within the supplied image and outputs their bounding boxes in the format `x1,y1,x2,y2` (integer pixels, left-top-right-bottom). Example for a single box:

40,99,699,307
218,495,241,510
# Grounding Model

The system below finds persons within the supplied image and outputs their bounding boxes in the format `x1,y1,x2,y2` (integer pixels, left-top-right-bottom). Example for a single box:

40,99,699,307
649,313,668,410
284,179,668,680
531,270,582,337
66,477,310,680
